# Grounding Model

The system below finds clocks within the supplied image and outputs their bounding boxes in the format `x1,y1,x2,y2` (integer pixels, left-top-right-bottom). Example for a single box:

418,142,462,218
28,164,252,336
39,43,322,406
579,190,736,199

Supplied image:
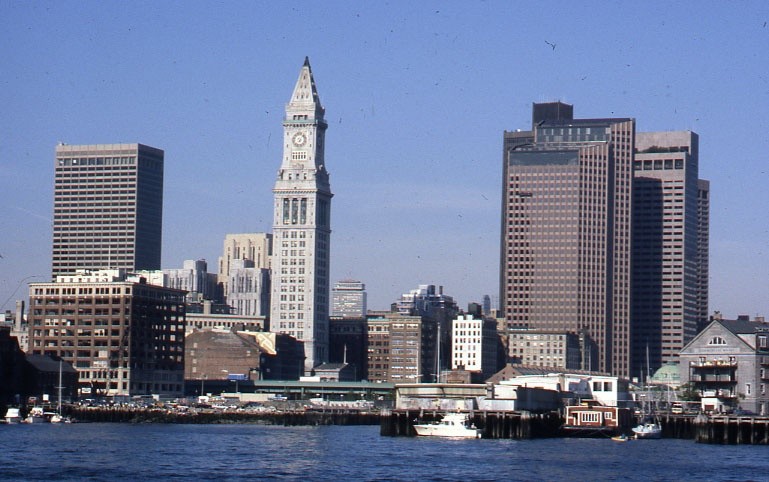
292,132,307,147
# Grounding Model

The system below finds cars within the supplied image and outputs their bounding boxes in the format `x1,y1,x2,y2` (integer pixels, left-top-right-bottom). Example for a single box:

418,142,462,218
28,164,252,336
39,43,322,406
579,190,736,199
83,400,91,406
688,408,698,415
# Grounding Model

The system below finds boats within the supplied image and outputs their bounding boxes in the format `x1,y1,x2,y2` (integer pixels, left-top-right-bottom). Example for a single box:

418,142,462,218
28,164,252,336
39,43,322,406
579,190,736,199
4,408,23,424
43,412,55,422
611,435,628,442
24,408,44,424
413,413,482,439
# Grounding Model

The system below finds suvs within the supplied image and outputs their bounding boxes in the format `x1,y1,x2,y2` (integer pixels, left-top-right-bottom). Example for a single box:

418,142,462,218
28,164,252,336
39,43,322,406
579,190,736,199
672,404,685,414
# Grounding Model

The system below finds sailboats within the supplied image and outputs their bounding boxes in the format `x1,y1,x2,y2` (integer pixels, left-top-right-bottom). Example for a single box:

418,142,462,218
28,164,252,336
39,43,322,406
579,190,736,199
631,344,662,438
50,360,66,423
